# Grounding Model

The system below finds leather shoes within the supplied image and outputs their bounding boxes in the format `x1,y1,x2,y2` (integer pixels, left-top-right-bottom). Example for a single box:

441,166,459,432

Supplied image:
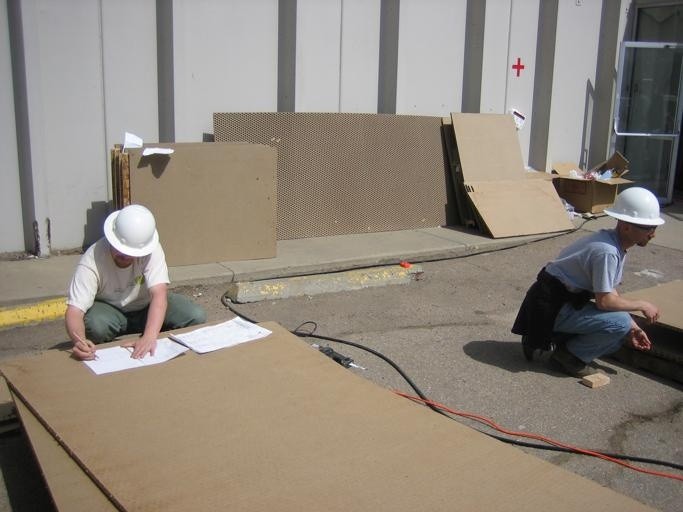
552,350,597,377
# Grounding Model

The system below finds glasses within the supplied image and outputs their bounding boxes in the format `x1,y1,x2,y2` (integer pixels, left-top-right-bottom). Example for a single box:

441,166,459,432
625,222,657,230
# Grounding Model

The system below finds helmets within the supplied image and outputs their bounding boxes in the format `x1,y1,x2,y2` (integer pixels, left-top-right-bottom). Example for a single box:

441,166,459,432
104,205,159,257
603,187,665,226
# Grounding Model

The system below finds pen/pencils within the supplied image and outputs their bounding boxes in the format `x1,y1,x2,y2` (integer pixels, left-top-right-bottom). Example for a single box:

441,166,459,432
72,331,99,358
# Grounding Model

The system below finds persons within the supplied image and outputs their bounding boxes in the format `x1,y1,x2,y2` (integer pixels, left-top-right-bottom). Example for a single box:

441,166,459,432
511,186,665,377
64,204,207,361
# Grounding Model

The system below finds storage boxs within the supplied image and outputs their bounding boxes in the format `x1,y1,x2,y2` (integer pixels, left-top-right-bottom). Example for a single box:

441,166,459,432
552,162,634,216
